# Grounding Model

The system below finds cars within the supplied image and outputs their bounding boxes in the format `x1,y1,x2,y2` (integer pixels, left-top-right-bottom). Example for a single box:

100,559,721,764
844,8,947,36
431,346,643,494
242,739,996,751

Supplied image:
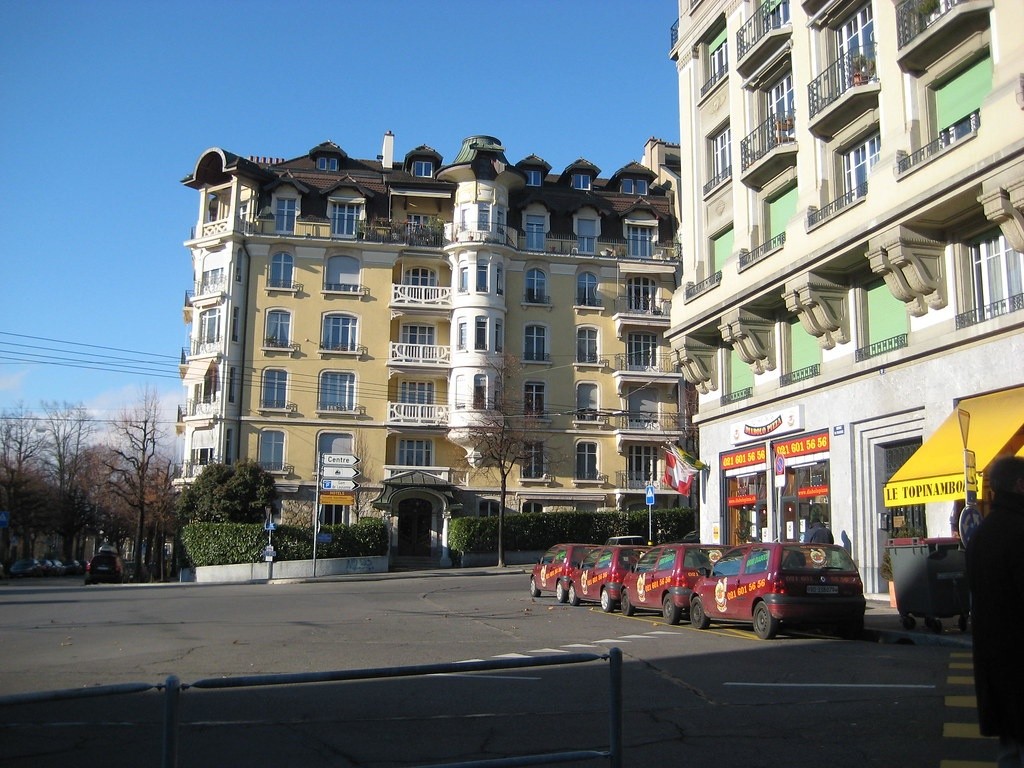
529,544,598,603
122,557,174,583
620,543,733,625
568,545,653,612
10,559,90,578
689,543,866,639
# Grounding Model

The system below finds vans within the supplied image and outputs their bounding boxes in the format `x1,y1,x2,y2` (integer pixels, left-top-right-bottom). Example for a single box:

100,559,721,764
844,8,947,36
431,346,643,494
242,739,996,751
604,536,646,545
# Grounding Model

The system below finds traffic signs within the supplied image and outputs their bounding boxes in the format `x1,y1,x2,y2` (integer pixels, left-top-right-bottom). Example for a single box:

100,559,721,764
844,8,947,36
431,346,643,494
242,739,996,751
321,453,361,491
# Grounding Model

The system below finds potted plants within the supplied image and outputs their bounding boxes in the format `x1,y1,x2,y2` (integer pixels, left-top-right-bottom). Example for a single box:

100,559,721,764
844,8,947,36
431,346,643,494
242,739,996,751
852,54,865,85
787,115,793,123
862,60,874,83
776,116,784,123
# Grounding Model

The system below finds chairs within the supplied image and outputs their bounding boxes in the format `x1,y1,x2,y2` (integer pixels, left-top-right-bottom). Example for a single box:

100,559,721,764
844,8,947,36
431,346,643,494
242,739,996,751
783,552,807,569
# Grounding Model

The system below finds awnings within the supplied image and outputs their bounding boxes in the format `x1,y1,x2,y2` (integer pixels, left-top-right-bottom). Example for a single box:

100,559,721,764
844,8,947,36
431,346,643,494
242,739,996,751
883,386,1024,507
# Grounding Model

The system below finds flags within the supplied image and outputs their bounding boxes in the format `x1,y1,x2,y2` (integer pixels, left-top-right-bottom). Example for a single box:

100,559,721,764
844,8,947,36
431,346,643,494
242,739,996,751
677,448,707,470
663,451,693,497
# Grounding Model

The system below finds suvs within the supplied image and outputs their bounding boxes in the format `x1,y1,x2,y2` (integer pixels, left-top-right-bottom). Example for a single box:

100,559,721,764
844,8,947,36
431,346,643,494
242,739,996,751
85,554,122,585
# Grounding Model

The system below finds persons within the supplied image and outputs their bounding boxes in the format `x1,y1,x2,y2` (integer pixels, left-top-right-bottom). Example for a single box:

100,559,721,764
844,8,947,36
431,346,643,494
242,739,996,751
803,518,834,546
966,457,1024,768
